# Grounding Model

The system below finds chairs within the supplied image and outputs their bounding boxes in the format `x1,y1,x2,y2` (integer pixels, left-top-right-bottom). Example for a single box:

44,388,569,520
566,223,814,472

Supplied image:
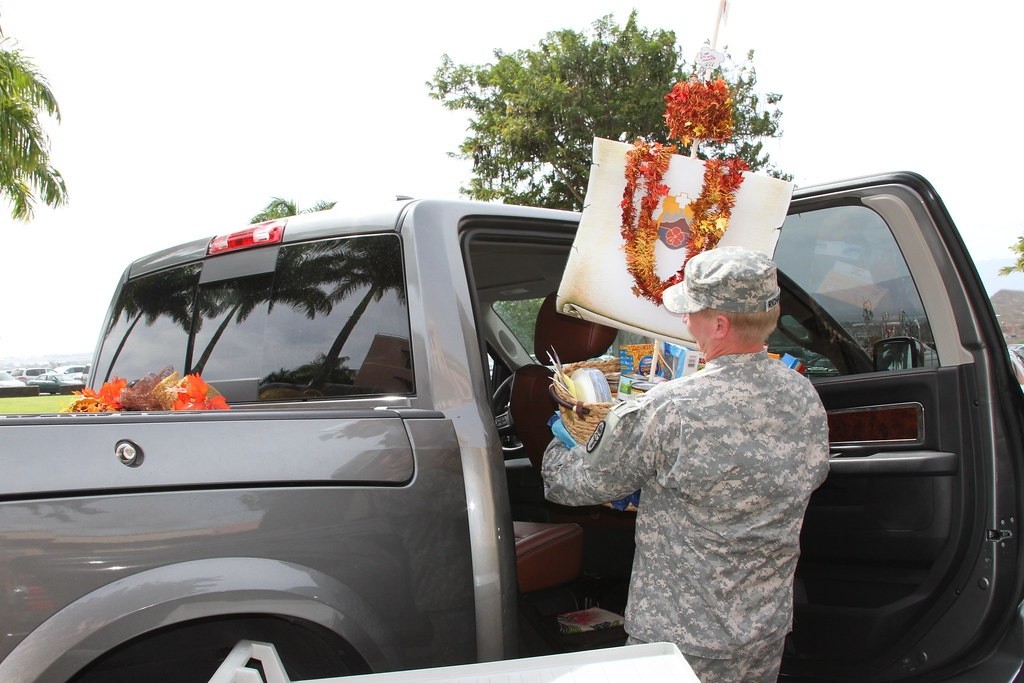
513,518,587,593
513,286,619,474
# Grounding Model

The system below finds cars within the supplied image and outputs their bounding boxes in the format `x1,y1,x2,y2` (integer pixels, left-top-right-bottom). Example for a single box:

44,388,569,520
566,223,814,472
0,369,28,387
794,337,1024,386
28,373,85,396
1,362,91,385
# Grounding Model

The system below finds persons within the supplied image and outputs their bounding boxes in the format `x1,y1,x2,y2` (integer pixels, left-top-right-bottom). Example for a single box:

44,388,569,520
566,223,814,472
539,246,832,683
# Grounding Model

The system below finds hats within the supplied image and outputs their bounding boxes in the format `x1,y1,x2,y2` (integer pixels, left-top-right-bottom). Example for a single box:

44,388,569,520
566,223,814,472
662,245,782,314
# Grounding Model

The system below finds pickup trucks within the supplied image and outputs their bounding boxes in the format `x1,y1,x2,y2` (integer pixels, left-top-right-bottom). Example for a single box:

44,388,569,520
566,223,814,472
0,185,1024,683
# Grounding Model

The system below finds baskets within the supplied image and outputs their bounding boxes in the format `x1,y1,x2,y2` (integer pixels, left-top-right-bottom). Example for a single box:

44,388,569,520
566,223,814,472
554,357,639,511
